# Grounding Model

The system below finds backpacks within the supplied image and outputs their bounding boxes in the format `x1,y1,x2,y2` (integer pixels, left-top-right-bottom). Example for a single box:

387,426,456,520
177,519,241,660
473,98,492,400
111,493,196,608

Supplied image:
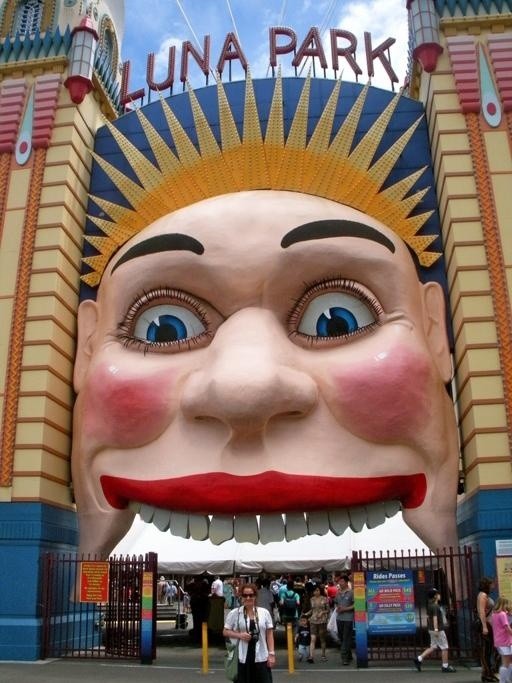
282,591,298,618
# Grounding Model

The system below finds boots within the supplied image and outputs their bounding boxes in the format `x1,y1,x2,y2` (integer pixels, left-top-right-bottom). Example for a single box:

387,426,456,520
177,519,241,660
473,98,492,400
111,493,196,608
499,663,512,683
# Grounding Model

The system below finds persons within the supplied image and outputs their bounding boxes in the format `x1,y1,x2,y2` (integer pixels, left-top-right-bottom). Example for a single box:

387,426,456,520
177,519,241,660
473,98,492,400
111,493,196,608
414,587,456,672
222,583,276,683
474,576,500,683
71,189,458,597
108,571,354,665
491,597,512,683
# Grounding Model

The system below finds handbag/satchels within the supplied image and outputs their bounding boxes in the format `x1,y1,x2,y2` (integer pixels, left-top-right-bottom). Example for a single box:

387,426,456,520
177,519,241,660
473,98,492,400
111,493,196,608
223,607,240,681
471,619,483,635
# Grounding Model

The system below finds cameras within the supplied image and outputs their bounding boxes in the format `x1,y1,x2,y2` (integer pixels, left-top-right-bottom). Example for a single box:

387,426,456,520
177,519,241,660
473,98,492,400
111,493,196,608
248,619,259,642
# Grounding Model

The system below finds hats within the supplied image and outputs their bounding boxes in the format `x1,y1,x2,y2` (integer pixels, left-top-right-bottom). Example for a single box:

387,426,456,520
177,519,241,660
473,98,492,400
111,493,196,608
159,575,165,580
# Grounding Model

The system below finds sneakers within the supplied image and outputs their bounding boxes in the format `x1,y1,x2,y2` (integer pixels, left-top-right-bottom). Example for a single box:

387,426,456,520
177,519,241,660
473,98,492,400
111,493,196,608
306,656,315,664
341,656,353,665
480,671,500,682
411,655,423,673
441,664,458,673
319,656,329,663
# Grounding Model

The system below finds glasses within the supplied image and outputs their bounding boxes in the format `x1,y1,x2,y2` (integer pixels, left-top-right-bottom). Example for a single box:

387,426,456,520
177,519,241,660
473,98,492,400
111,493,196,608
240,593,257,599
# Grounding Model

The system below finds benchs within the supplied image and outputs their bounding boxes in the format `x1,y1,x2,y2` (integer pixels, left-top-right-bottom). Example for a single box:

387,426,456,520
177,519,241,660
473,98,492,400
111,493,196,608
99,604,180,632
